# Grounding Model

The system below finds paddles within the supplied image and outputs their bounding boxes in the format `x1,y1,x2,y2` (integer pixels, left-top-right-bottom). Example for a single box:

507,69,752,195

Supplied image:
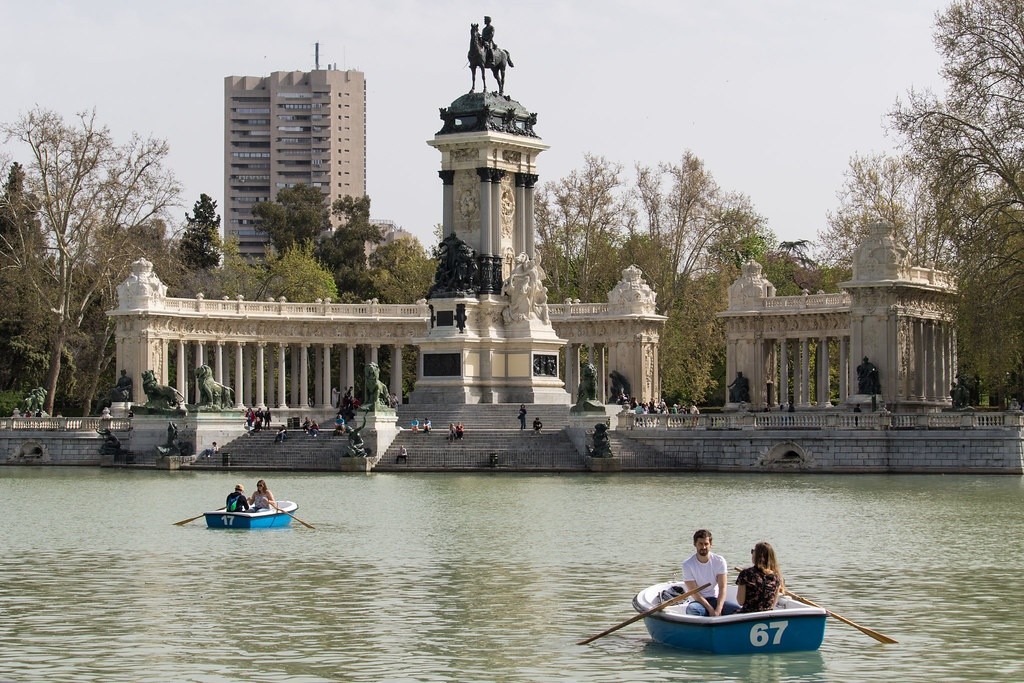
262,497,315,529
734,567,898,644
577,582,710,645
173,497,250,525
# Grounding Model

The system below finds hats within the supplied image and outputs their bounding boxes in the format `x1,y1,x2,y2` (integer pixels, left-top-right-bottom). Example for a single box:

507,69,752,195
234,484,244,492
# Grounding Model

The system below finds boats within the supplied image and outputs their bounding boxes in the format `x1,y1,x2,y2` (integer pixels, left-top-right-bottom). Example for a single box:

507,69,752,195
632,578,827,654
203,500,299,529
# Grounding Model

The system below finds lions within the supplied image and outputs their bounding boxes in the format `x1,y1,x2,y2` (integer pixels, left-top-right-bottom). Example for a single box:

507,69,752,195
193,365,234,408
365,361,391,408
140,370,185,407
577,362,597,400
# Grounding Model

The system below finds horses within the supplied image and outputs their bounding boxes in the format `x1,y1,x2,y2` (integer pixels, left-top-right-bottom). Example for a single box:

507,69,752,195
467,23,514,96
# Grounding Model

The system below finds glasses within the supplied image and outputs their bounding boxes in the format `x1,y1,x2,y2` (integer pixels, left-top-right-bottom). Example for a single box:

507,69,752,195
258,485,262,487
751,549,755,553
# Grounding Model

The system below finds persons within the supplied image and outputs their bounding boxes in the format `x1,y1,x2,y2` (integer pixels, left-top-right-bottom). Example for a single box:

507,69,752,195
482,16,495,66
394,417,464,464
96,429,120,449
728,372,748,402
735,542,782,613
246,386,359,443
682,529,741,616
859,357,880,393
226,479,275,513
24,411,63,426
519,405,543,434
168,422,177,446
206,441,216,458
346,417,367,458
854,403,862,427
636,399,697,424
438,232,477,289
108,369,132,401
514,260,549,323
789,404,795,425
